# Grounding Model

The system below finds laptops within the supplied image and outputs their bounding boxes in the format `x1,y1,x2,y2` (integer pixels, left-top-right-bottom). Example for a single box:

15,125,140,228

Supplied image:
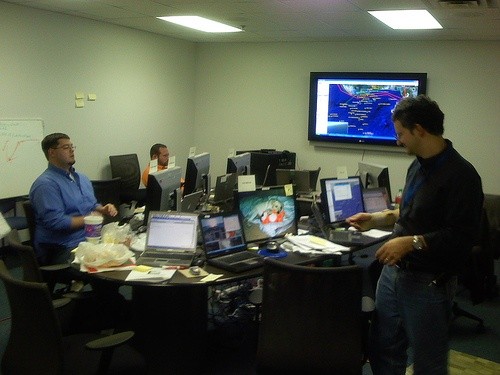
135,211,201,267
198,210,273,274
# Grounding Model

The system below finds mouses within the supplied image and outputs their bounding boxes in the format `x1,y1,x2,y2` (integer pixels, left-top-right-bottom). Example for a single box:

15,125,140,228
189,267,201,276
388,203,396,209
267,243,278,252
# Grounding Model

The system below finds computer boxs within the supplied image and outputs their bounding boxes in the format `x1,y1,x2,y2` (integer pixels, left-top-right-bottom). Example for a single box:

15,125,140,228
236,151,296,188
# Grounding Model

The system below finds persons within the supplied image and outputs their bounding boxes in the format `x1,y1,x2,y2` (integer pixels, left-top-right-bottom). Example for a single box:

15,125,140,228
29,133,118,280
345,94,483,375
142,143,185,188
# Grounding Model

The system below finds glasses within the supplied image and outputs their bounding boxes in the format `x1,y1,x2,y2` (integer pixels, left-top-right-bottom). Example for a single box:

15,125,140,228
53,144,77,151
392,125,416,142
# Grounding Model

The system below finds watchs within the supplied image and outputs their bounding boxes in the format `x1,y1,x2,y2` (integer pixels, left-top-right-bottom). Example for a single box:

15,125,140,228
412,235,424,251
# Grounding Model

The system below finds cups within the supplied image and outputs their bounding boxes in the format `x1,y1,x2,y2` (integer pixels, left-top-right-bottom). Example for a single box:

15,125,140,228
86,236,101,244
83,216,103,236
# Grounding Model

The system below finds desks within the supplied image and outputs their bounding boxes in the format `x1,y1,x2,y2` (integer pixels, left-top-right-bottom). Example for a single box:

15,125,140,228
65,189,399,375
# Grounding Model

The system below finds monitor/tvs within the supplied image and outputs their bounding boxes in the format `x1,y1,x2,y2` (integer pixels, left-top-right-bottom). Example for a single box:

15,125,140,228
142,167,183,223
357,160,393,204
238,187,297,245
320,176,365,228
226,149,251,192
184,151,213,206
308,71,428,146
360,187,390,214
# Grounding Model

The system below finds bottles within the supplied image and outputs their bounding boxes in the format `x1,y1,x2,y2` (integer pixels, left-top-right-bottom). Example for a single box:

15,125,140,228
297,215,313,235
395,189,403,208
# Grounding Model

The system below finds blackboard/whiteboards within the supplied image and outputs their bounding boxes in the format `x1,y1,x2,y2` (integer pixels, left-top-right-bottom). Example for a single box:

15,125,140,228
0,119,48,200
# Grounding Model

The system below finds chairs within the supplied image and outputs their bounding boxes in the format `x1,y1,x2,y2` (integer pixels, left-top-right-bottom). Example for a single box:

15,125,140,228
0,153,500,375
